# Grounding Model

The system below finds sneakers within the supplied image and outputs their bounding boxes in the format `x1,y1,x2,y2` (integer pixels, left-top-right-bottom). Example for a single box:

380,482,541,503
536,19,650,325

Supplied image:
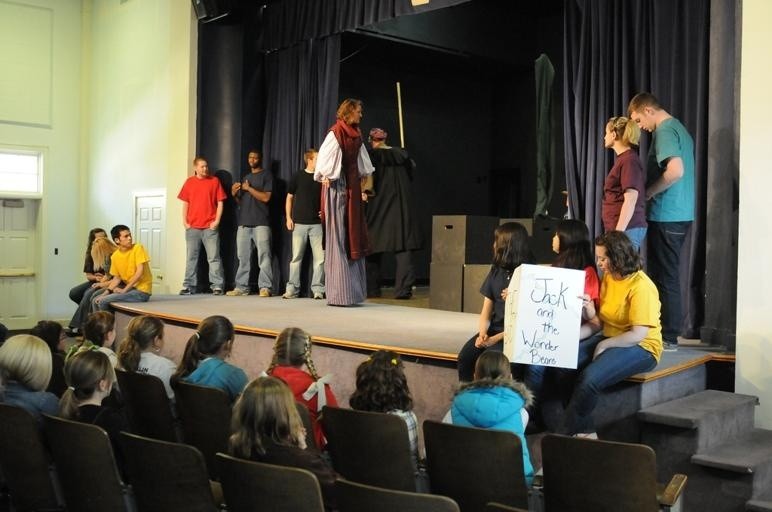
213,288,223,295
283,291,299,299
180,287,195,295
259,288,271,297
226,290,249,296
75,335,84,342
314,293,323,299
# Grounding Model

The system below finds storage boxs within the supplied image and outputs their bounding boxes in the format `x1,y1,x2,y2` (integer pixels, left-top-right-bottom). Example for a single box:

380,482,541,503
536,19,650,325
464,264,494,313
500,219,557,259
429,261,462,311
432,216,494,263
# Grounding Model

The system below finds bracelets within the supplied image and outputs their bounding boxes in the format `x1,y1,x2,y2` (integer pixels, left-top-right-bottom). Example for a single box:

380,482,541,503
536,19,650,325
107,288,112,294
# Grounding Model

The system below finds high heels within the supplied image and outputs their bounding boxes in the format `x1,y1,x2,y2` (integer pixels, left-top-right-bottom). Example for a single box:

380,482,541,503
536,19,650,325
65,328,82,337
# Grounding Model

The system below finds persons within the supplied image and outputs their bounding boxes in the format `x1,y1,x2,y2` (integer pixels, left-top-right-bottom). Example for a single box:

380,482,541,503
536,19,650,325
457,222,537,382
65,227,108,304
440,351,535,488
228,376,340,511
92,227,153,315
0,333,61,435
118,314,178,402
30,321,68,387
58,350,127,480
348,347,419,476
364,128,416,300
313,98,375,305
282,148,325,299
262,326,338,450
225,147,274,297
178,156,228,296
601,117,648,251
536,231,662,478
168,315,249,402
501,219,600,392
65,237,119,337
627,92,694,351
65,312,122,392
